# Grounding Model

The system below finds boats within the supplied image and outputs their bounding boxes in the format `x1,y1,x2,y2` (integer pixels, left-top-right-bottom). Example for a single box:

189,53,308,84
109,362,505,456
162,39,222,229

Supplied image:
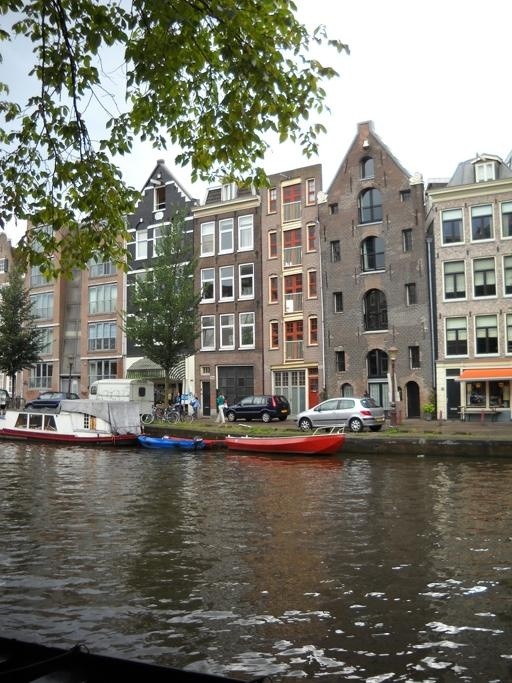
138,435,225,450
0,411,139,446
224,434,345,456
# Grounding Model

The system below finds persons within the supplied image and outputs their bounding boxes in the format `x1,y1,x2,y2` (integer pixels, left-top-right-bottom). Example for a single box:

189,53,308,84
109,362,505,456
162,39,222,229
318,388,327,403
175,393,180,403
214,391,227,424
192,397,200,419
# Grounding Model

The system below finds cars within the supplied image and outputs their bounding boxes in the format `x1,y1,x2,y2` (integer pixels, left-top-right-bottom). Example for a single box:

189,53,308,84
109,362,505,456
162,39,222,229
224,395,290,423
24,392,79,410
296,397,385,432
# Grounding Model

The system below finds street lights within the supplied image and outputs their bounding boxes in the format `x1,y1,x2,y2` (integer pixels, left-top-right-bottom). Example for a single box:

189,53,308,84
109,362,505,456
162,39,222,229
387,346,399,426
68,355,74,397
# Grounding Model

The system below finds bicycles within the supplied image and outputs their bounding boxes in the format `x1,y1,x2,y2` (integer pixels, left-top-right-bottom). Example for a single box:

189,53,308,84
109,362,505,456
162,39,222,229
141,405,194,425
